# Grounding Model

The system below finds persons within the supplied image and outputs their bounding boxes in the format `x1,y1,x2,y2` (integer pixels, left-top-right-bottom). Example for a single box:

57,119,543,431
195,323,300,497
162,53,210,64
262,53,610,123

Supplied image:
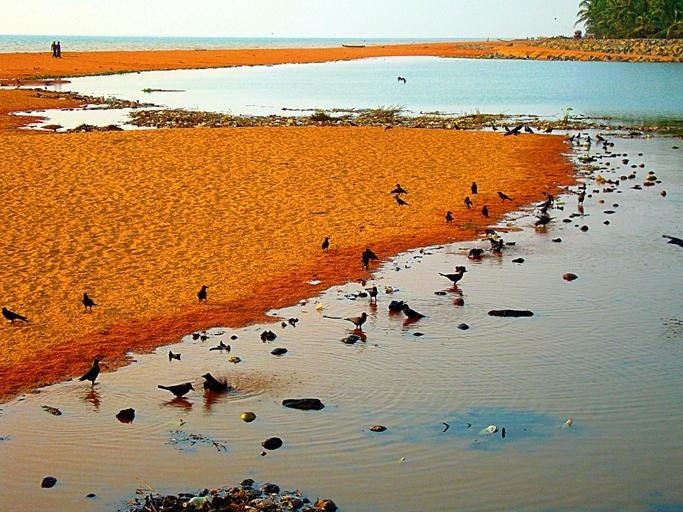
51,41,56,56
56,41,60,57
574,32,579,40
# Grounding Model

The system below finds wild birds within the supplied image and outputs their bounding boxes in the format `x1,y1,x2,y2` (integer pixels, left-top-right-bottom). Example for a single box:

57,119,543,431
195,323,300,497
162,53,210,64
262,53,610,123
196,284,208,303
320,236,330,251
156,372,228,397
344,249,466,331
78,356,101,387
81,291,95,313
1,307,28,325
397,76,405,83
388,181,587,259
490,121,610,150
